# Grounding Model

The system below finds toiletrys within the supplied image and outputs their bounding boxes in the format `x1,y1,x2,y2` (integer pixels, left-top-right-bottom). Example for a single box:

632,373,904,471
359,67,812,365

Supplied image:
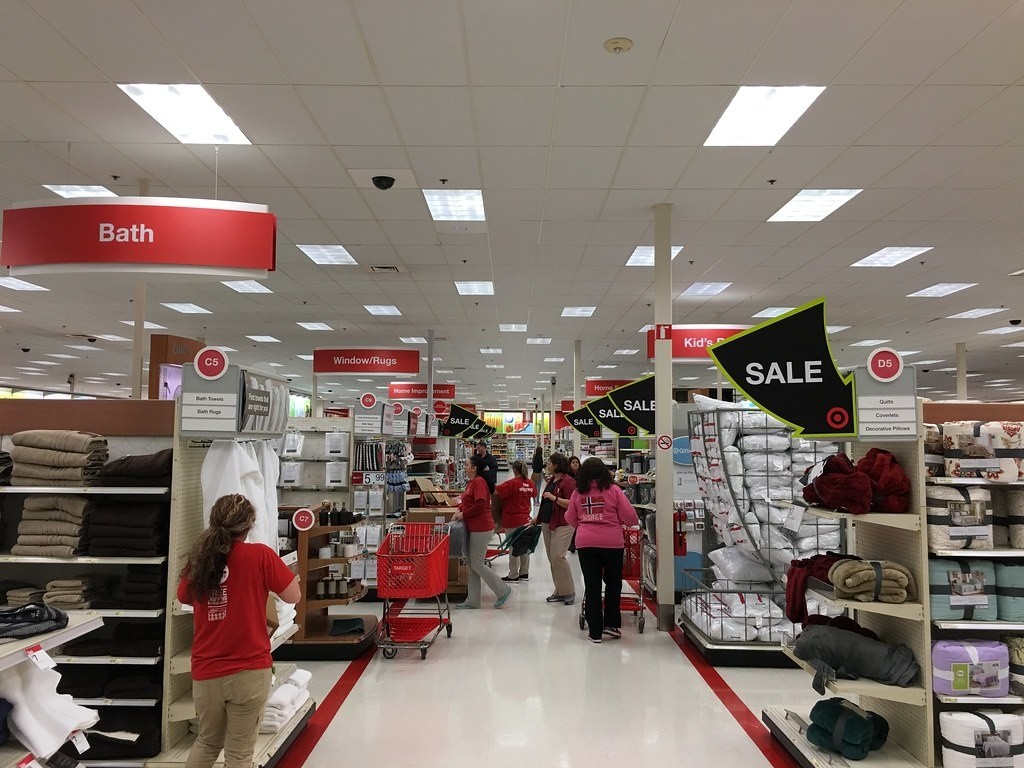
316,576,325,600
319,502,328,526
330,501,349,526
339,573,348,599
328,574,337,599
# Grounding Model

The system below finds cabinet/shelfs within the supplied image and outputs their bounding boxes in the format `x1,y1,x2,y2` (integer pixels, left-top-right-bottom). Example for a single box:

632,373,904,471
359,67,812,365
0,364,1024,768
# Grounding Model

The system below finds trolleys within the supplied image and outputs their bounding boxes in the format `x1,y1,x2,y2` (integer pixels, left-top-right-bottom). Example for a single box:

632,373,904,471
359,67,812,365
374,522,452,660
578,524,645,634
484,524,536,568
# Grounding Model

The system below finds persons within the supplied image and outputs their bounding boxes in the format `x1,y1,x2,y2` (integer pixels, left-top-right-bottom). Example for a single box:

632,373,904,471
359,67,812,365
471,442,497,496
568,456,581,553
452,456,512,610
496,460,538,583
530,447,545,505
177,493,301,768
564,458,638,643
530,453,577,605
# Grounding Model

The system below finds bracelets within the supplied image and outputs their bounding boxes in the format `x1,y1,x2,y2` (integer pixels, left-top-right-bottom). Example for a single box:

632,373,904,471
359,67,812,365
553,496,558,503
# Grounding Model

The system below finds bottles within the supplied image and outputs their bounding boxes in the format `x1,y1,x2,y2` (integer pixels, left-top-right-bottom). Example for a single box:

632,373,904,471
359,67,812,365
340,502,348,526
318,502,329,526
330,502,340,526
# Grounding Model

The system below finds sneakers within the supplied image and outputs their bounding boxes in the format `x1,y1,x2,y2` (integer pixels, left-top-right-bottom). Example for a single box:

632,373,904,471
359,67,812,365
603,626,622,636
455,599,481,608
546,594,565,602
518,574,528,581
501,576,519,583
587,633,603,643
494,587,513,608
564,592,576,605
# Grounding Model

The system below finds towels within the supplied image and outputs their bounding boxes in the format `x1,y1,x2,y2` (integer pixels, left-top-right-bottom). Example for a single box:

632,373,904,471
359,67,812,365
0,655,101,760
98,448,172,486
0,602,69,640
6,587,44,607
64,707,164,761
103,664,164,700
90,494,170,557
109,563,168,610
10,493,89,557
327,618,365,637
42,578,106,610
256,668,313,736
200,439,280,602
8,428,110,487
112,619,164,657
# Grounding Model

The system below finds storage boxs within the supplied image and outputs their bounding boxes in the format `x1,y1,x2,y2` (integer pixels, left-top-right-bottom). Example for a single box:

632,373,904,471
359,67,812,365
406,507,460,587
